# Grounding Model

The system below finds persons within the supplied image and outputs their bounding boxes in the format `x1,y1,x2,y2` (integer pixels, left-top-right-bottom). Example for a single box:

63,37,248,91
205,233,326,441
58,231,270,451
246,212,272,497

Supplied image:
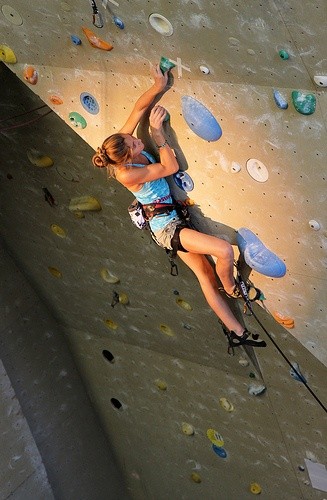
92,63,267,356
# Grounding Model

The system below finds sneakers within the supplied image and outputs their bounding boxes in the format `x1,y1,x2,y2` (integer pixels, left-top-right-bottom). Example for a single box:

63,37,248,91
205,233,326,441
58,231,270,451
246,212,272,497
226,281,261,301
229,328,266,348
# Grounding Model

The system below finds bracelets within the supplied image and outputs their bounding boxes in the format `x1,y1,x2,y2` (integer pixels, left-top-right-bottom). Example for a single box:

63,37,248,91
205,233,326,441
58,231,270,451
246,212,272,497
156,140,167,148
